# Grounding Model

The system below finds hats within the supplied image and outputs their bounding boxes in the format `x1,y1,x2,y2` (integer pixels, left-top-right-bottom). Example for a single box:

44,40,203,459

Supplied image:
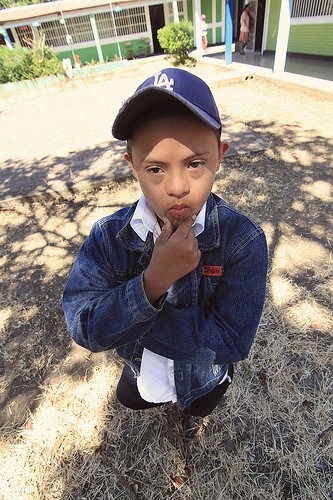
112,68,222,140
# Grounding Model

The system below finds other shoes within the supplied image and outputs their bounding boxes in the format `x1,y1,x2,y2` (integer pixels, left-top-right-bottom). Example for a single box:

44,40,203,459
188,415,202,432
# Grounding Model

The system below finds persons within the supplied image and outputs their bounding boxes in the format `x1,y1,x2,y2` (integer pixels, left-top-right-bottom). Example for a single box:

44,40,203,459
201,14,208,51
62,68,268,497
239,4,251,54
